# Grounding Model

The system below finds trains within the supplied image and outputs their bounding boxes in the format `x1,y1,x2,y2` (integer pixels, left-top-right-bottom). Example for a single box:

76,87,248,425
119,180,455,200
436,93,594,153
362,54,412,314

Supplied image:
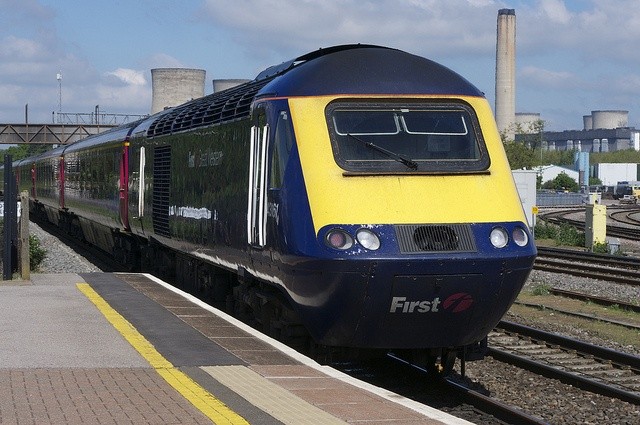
0,43,539,375
590,186,640,199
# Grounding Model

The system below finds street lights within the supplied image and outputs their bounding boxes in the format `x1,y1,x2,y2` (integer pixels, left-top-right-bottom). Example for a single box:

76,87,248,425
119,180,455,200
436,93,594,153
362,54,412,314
57,73,62,123
